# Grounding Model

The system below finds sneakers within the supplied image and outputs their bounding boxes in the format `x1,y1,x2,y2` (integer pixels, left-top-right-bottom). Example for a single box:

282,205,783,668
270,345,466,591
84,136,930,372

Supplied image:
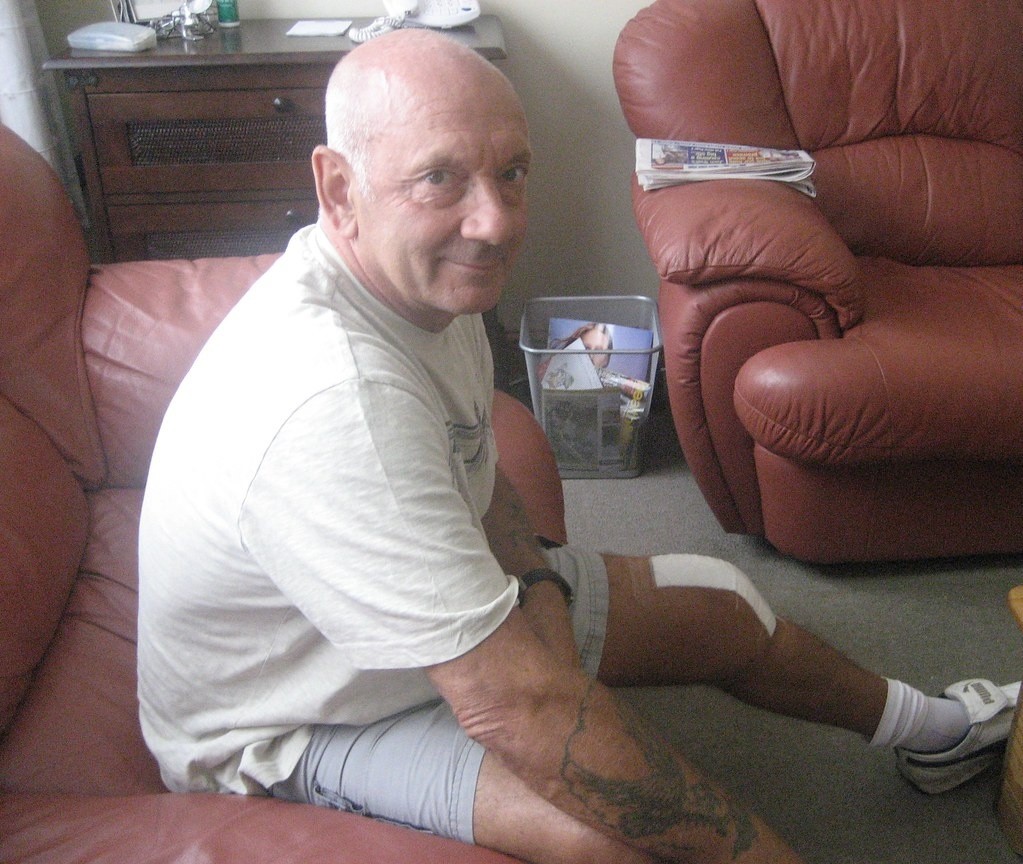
892,678,1020,794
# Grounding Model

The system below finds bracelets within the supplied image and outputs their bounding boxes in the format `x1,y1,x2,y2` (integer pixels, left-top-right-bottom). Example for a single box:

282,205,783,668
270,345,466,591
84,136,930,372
518,568,574,609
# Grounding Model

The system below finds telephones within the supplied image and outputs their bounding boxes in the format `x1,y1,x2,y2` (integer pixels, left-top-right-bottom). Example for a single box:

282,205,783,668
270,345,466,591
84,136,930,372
382,0,482,30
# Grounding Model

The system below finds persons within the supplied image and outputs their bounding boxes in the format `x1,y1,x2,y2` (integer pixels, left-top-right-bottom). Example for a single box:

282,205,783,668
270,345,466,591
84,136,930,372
563,323,613,368
136,29,1023,864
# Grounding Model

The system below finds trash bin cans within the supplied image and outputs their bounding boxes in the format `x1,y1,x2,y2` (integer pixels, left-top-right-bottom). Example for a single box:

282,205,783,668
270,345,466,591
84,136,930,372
517,293,666,481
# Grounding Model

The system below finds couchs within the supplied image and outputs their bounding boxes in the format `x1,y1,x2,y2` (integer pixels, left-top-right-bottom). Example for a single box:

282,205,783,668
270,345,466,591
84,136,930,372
0,121,567,862
612,1,1023,564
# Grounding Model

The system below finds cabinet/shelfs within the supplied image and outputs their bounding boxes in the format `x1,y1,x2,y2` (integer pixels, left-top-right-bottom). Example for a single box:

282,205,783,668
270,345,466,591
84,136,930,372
42,12,508,267
